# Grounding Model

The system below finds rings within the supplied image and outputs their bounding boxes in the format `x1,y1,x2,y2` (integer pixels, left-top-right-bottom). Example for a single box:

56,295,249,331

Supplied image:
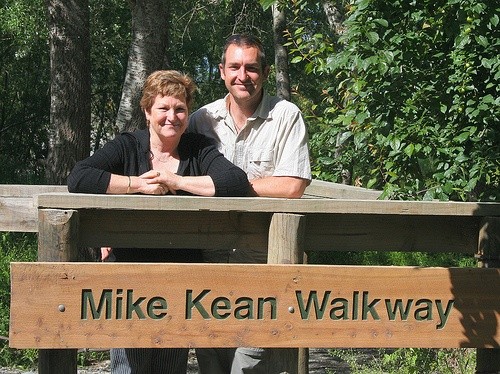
156,171,160,176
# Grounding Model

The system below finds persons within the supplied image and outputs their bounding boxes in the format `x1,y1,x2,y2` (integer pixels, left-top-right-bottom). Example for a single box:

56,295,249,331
182,31,312,374
64,72,250,373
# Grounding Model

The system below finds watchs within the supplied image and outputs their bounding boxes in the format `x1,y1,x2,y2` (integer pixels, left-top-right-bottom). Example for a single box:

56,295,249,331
126,175,132,195
